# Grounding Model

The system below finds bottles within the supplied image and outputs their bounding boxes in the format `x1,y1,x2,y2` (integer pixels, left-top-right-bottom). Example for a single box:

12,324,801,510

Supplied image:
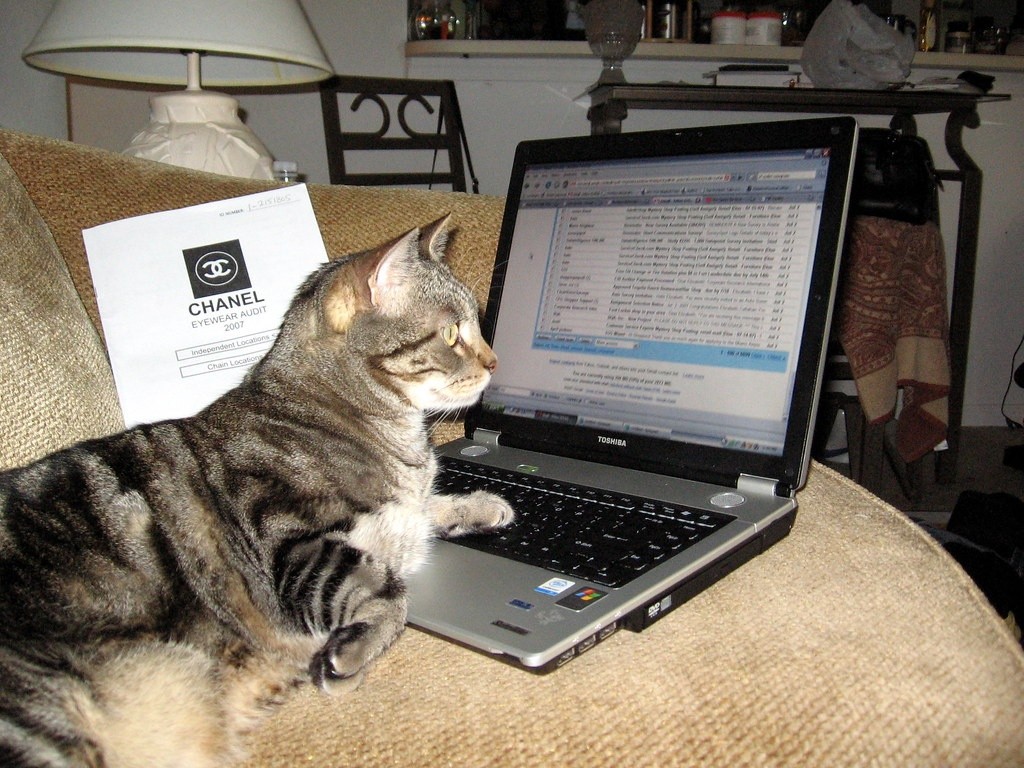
919,0,937,53
272,161,299,183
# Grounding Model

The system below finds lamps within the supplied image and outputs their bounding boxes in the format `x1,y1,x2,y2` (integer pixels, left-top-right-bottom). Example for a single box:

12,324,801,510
23,0,336,180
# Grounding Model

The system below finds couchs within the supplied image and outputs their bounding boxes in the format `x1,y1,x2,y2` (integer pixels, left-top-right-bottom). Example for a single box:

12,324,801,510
0,132,1024,768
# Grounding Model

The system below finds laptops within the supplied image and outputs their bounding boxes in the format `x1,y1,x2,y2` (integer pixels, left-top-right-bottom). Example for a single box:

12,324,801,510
405,116,860,676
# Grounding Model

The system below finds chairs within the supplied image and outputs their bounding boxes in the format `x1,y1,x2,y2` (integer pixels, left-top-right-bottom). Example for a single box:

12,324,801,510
588,83,1012,453
320,72,478,192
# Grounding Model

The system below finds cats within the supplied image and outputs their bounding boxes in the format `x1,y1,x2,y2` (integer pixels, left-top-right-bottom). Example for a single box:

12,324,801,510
0,212,514,768
